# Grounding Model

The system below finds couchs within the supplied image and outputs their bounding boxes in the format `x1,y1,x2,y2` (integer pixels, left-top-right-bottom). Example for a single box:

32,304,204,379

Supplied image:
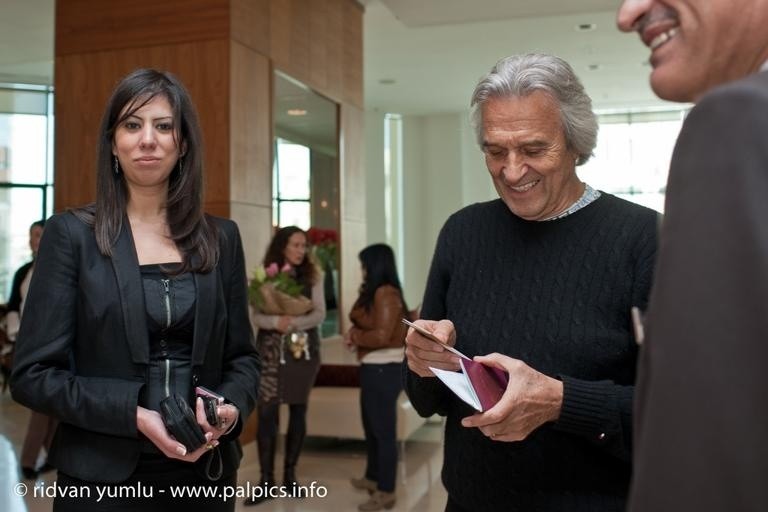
281,334,443,458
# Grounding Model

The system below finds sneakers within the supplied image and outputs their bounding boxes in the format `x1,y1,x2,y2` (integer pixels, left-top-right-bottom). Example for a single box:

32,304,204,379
20,459,53,480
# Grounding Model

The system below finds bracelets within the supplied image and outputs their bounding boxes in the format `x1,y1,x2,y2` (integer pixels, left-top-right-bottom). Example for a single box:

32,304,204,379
221,401,241,438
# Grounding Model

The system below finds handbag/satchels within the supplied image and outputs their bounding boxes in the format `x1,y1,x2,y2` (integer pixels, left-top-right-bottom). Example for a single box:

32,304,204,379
0,318,17,376
276,357,323,405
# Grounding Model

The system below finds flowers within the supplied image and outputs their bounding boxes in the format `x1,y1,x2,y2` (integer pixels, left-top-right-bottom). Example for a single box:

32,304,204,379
306,227,339,260
246,263,303,313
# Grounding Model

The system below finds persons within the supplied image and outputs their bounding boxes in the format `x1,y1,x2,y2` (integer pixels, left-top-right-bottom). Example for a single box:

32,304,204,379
616,1,768,511
339,238,414,511
244,224,330,504
0,216,66,479
403,48,668,511
7,68,266,511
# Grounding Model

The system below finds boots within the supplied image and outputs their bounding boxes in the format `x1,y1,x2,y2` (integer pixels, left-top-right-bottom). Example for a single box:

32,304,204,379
282,432,308,499
243,430,277,506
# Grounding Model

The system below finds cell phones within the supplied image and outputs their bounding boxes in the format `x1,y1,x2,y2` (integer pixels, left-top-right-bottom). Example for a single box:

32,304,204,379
195,386,226,430
196,393,216,425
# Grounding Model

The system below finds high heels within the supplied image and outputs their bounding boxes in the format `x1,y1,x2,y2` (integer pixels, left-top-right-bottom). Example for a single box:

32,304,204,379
347,473,379,491
358,488,397,510
